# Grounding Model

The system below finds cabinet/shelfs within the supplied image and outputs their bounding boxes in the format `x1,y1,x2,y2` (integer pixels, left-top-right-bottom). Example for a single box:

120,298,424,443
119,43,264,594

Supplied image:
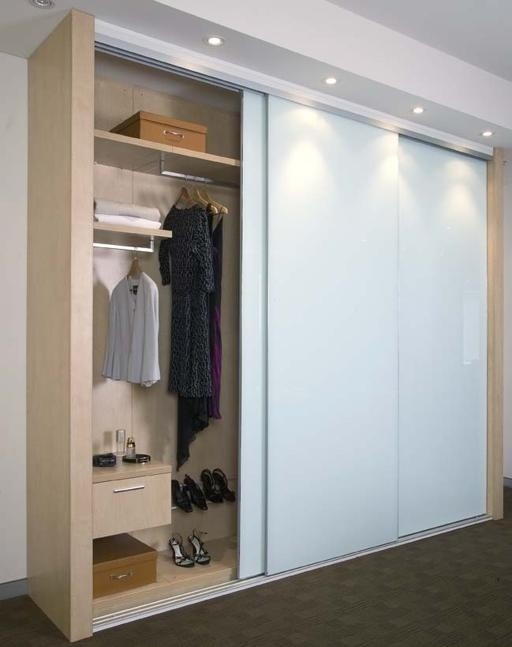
27,8,509,642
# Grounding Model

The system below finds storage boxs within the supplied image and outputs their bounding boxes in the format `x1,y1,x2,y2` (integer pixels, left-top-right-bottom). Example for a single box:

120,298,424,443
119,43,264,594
93,533,157,598
110,111,207,153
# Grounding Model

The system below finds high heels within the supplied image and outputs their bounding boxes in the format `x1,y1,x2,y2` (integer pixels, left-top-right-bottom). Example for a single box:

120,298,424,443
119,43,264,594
187,527,211,564
166,531,196,569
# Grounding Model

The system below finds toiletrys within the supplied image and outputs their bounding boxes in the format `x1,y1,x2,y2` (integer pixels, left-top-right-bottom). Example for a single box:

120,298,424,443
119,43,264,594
126,443,135,457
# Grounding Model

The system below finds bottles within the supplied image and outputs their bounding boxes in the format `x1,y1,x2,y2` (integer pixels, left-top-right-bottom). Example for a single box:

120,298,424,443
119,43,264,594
126,437,136,457
116,430,125,455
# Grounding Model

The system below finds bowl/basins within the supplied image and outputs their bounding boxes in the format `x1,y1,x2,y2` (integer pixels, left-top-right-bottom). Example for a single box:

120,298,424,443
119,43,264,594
94,454,116,467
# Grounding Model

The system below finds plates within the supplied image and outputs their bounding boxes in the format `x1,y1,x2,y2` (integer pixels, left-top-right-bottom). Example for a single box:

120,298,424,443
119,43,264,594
123,454,150,463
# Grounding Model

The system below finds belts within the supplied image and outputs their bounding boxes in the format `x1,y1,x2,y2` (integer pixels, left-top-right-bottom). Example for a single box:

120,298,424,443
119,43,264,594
120,453,153,464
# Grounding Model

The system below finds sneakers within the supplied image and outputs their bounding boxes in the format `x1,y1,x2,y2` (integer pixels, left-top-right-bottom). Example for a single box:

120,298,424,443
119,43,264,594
212,468,239,503
199,467,225,504
182,472,210,511
170,479,194,513
